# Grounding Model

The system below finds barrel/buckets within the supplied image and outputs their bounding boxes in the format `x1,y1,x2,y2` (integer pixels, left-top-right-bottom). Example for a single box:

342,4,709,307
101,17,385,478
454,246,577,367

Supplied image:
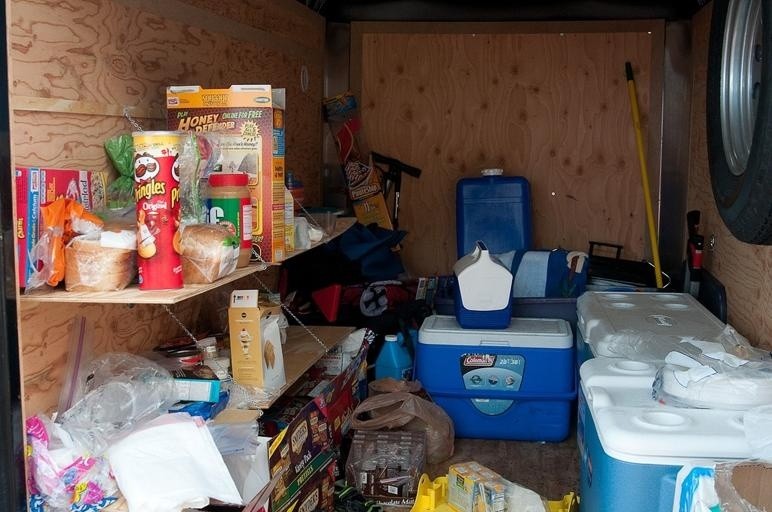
455,167,535,276
374,332,413,381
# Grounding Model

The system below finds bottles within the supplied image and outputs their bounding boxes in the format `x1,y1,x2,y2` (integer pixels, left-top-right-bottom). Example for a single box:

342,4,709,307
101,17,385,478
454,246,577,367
376,334,411,380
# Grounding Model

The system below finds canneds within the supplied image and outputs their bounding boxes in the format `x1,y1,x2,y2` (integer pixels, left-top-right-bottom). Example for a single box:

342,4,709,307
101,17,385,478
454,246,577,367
168,349,203,371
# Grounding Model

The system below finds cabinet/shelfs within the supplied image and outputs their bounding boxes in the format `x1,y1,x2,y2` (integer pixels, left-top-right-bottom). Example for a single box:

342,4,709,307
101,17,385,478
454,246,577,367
17,211,357,445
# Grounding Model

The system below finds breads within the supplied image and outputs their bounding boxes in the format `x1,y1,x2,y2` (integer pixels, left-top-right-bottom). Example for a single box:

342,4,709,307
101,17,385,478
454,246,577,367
181,225,232,285
64,239,135,293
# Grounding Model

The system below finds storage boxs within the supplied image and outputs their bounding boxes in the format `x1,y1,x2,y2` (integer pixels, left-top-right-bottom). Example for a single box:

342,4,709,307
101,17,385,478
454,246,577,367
17,163,108,293
570,359,772,512
417,313,577,443
206,340,368,511
165,80,284,266
225,288,286,393
571,286,734,370
